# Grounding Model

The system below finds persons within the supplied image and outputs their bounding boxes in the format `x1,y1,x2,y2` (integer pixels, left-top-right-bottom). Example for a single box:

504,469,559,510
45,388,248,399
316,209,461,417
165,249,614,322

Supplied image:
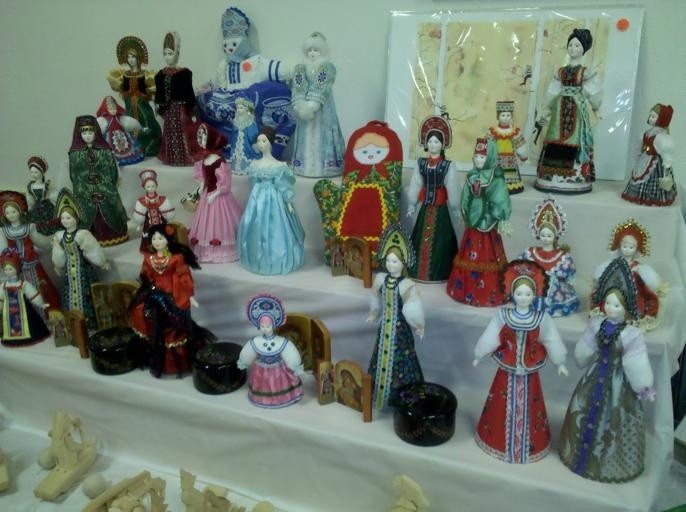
0,154,112,348
446,141,513,306
472,196,580,464
620,102,678,206
485,100,529,195
407,114,460,282
533,28,603,196
136,226,201,369
237,295,306,408
0,6,678,485
364,223,425,410
559,218,670,483
68,115,130,246
96,8,346,177
134,123,306,275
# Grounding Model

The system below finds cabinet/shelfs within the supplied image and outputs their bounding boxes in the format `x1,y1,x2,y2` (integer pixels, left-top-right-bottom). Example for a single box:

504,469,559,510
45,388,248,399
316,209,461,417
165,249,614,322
0,151,686,512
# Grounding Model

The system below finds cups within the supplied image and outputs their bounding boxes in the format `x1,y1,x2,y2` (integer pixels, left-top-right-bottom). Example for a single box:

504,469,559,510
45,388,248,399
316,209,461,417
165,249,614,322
396,381,457,447
260,97,294,128
86,326,142,375
193,341,245,394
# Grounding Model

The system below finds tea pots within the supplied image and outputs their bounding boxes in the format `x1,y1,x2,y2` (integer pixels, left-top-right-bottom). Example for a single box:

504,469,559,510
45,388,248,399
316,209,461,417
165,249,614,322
199,86,253,127
